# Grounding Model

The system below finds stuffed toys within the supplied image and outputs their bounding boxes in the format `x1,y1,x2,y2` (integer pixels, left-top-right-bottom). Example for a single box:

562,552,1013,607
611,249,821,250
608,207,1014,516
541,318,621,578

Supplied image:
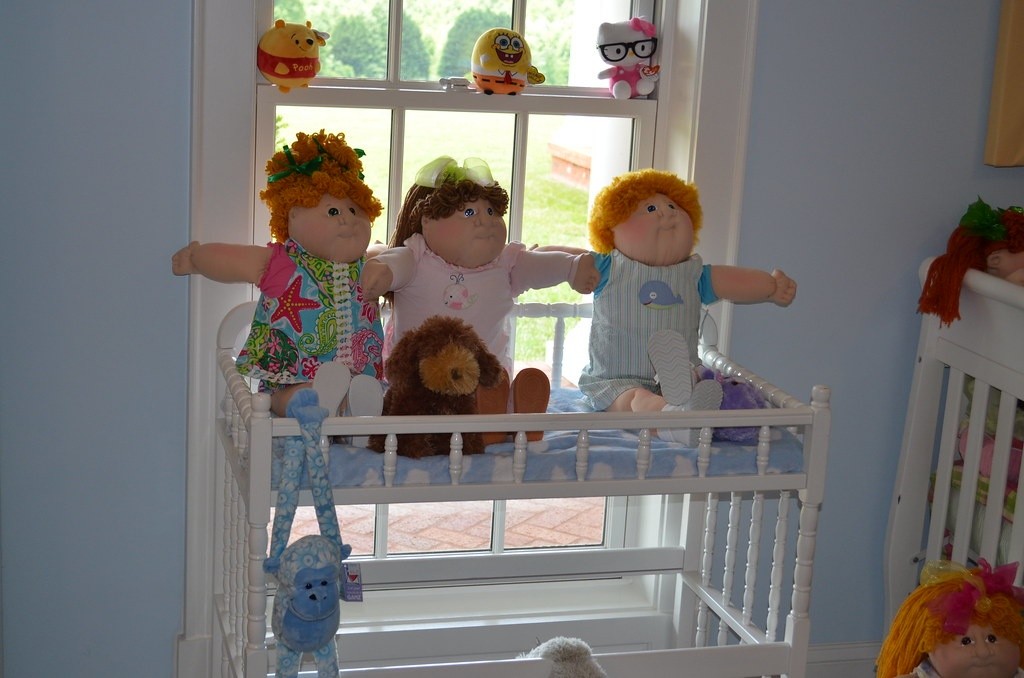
530,168,798,448
172,130,386,416
918,201,1024,330
596,14,660,100
367,312,502,459
957,375,1024,481
260,386,352,678
362,156,601,441
872,556,1023,677
514,637,606,678
468,28,545,95
257,20,331,94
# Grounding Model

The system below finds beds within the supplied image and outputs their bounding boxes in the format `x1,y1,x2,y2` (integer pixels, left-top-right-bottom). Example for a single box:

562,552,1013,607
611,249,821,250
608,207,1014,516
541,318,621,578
204,297,836,678
882,256,1024,649
